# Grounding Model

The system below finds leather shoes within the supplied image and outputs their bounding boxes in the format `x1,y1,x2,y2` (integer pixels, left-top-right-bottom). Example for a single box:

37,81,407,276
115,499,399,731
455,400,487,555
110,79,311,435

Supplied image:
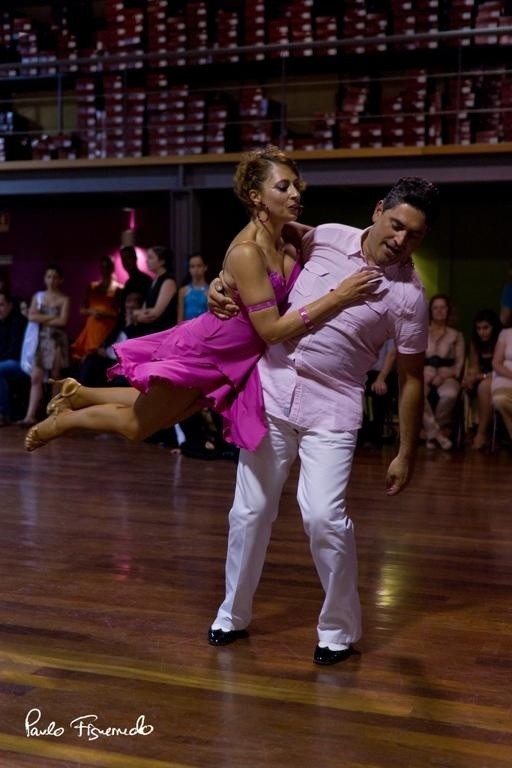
313,640,352,664
208,623,246,646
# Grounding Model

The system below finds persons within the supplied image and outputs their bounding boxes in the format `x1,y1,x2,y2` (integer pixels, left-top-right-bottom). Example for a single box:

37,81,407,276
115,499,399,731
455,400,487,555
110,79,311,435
24,141,420,450
2,228,512,463
206,175,442,668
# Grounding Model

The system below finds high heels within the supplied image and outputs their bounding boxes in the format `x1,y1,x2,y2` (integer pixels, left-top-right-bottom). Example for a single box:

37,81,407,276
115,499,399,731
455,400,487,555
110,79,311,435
24,406,72,451
46,377,81,414
